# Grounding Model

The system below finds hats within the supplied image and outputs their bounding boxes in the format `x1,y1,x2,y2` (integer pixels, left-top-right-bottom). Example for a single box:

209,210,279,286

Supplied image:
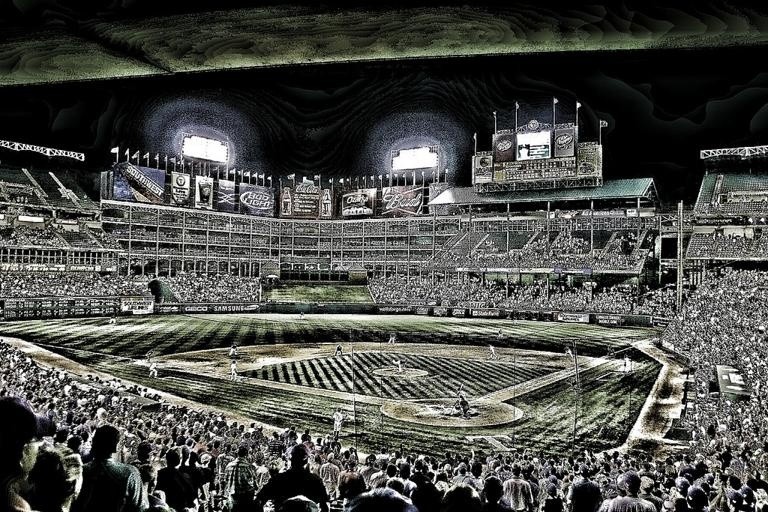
662,472,753,507
579,464,590,473
200,453,212,463
0,395,57,449
458,462,468,471
327,452,335,461
137,442,157,455
472,462,483,470
368,454,376,462
596,473,655,497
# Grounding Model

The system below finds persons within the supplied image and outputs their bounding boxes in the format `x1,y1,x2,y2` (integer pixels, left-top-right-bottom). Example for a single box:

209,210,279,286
0,194,768,512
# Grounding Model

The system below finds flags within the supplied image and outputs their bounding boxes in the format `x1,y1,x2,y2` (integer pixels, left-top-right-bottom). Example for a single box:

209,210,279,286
493,111,497,118
472,133,476,140
554,98,559,105
516,103,520,109
600,120,608,128
577,103,582,108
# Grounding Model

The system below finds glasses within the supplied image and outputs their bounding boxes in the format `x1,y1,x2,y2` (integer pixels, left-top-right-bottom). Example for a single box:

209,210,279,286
22,438,46,448
